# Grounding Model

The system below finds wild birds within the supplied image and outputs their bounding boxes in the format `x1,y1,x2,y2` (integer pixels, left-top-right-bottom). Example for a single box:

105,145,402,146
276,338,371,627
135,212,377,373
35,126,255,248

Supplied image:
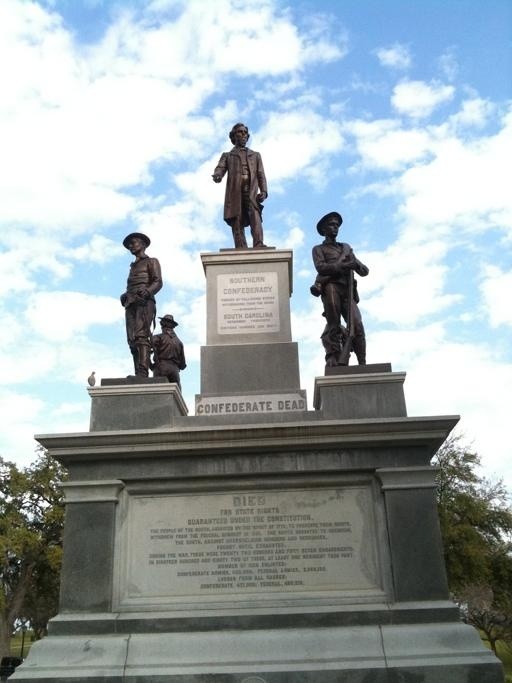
88,371,96,386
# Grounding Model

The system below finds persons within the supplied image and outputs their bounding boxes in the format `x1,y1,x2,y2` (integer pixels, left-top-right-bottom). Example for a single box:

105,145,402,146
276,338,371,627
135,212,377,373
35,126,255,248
309,211,369,365
321,319,354,366
147,314,186,391
120,231,163,378
210,123,268,248
